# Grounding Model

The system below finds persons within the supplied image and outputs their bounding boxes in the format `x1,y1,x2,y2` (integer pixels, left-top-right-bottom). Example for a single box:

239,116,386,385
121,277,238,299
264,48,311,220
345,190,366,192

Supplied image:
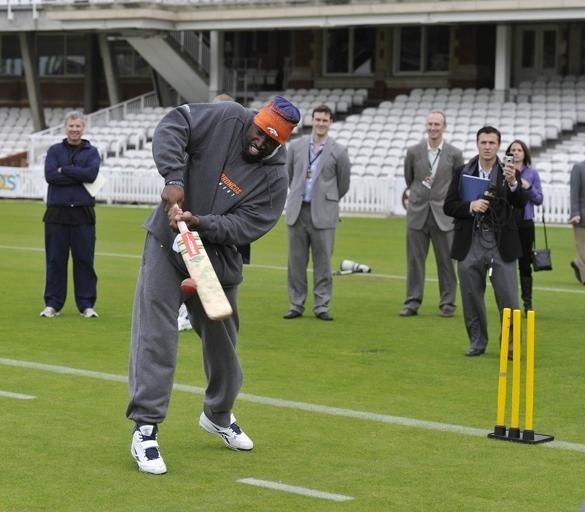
128,94,301,476
505,140,544,317
39,111,101,319
283,106,351,321
399,111,465,318
443,126,529,360
569,162,584,284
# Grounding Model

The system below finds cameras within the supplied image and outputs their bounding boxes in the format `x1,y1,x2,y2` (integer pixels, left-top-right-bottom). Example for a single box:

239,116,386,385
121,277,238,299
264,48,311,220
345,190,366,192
505,156,513,168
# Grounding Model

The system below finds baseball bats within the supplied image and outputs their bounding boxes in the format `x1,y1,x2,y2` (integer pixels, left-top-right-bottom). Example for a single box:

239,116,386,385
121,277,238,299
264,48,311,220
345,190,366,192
171,202,234,321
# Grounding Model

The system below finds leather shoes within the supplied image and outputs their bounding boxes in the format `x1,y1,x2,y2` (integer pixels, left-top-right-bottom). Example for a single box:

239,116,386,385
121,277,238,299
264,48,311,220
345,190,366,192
400,308,417,317
282,310,300,320
439,305,456,317
569,259,581,283
507,350,522,361
318,312,332,322
465,347,485,357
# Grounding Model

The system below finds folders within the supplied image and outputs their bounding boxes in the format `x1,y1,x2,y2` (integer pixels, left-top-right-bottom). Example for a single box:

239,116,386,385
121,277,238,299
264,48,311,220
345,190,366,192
462,174,491,202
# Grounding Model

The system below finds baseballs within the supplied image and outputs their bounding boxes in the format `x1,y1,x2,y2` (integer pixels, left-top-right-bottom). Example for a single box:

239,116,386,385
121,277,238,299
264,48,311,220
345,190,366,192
181,278,196,293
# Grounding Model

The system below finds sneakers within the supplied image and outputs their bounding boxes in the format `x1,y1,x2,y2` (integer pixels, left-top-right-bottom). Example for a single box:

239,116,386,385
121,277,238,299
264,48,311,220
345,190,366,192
79,307,99,319
196,411,253,452
178,304,192,334
39,306,61,318
129,424,168,475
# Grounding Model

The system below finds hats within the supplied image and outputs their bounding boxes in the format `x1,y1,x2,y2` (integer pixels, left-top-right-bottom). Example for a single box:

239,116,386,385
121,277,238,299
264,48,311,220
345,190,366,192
250,94,301,145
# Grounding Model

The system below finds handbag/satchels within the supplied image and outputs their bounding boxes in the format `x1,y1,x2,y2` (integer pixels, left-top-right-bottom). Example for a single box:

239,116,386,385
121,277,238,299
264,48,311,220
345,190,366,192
532,248,554,271
